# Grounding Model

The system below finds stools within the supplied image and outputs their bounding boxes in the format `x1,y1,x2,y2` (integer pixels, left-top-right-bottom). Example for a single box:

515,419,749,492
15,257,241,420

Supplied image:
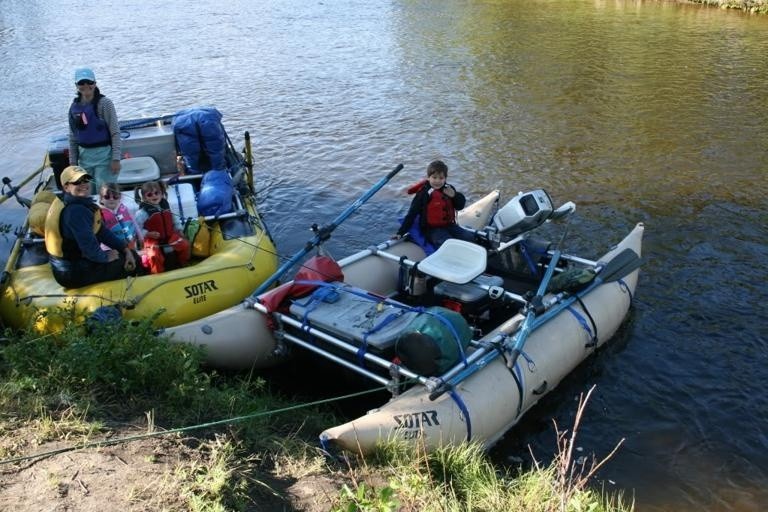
419,238,491,285
115,155,160,184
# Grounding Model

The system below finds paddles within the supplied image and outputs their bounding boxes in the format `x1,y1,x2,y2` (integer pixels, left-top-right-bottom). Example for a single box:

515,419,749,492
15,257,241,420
428,248,643,401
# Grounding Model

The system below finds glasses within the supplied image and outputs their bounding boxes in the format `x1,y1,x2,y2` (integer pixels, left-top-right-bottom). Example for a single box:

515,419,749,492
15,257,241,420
77,79,94,85
145,190,161,198
102,194,121,200
70,177,90,186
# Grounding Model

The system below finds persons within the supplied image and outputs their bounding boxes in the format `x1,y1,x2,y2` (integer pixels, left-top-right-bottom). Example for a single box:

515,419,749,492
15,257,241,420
44,167,147,289
387,159,477,254
132,179,192,275
67,68,123,195
96,181,145,256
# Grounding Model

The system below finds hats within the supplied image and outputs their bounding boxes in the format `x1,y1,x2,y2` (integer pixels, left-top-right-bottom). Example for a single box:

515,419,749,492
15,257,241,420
74,67,96,83
60,165,93,185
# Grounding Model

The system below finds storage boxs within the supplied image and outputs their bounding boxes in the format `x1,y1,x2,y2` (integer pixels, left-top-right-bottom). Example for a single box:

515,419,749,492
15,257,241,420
431,274,504,340
89,185,198,238
289,280,420,383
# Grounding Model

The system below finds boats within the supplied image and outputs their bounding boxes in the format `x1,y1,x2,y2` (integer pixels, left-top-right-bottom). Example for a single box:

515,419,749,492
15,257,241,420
0,107,278,347
151,191,645,465
